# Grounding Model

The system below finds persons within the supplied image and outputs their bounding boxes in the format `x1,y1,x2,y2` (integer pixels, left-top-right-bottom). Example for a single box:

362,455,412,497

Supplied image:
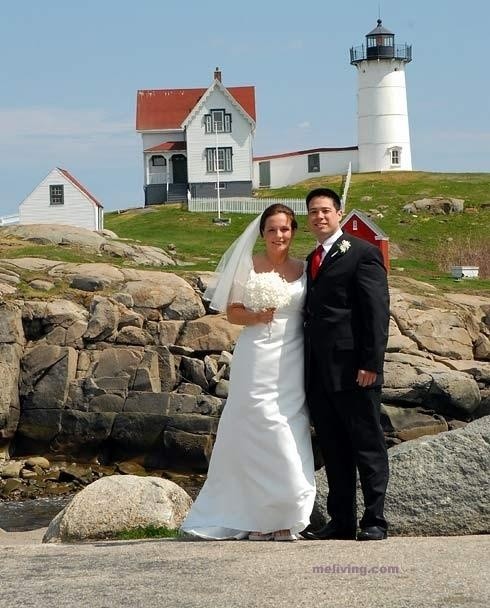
181,188,391,540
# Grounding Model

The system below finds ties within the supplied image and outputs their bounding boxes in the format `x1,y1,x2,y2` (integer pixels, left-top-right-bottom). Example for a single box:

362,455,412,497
310,245,324,280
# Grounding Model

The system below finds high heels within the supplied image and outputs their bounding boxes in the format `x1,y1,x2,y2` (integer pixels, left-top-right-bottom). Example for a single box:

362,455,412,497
248,530,294,541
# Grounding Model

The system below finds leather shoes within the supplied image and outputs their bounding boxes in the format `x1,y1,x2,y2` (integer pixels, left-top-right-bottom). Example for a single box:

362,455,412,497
299,520,388,540
